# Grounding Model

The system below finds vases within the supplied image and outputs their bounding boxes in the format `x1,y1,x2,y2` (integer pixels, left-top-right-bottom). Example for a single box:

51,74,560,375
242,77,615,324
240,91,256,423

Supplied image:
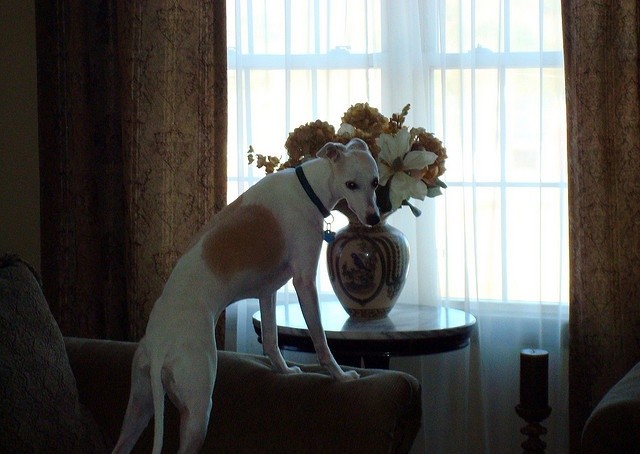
325,208,409,321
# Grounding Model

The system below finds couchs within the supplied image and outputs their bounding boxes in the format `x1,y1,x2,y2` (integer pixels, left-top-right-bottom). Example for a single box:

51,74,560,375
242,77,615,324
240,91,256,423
1,253,421,454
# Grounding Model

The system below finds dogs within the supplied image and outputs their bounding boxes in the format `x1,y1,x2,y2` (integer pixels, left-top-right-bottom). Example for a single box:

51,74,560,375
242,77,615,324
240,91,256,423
113,137,382,449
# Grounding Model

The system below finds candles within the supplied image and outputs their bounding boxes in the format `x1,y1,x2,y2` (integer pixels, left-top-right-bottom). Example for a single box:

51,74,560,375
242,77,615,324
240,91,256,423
520,349,550,411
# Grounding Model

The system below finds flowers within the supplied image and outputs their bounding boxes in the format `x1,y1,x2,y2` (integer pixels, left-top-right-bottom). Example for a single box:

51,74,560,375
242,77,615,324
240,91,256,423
247,103,448,217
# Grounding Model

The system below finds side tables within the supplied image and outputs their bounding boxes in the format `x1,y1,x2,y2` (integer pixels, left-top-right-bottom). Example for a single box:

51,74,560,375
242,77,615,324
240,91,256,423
252,301,477,368
